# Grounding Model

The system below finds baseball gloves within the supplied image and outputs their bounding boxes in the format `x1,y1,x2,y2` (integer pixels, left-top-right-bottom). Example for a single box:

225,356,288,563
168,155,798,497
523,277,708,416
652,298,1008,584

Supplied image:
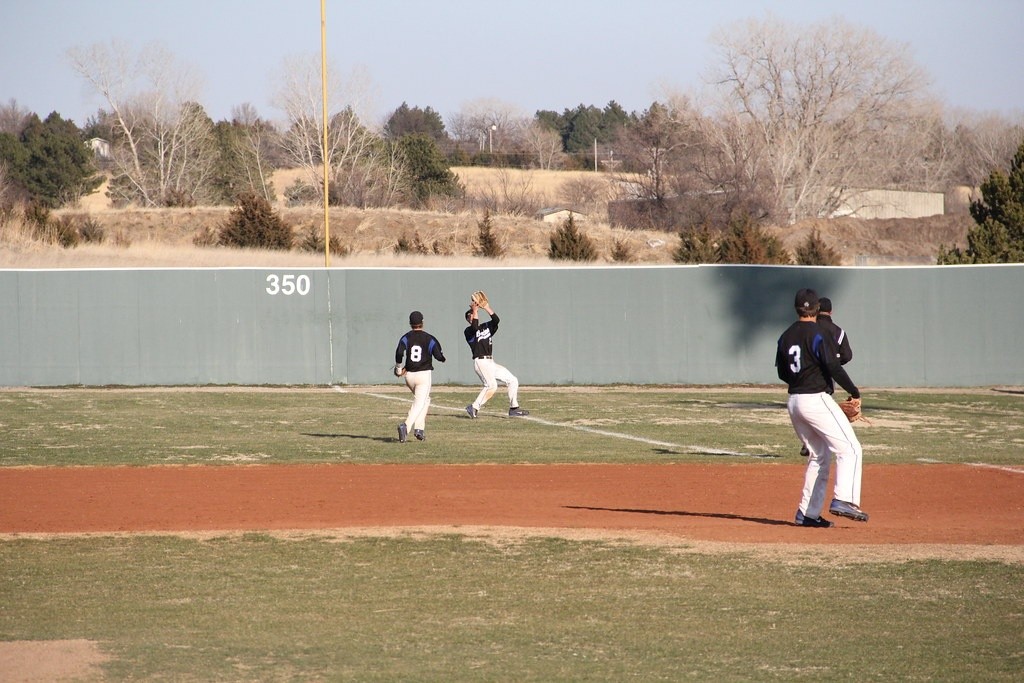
388,364,406,378
471,290,488,309
839,396,873,427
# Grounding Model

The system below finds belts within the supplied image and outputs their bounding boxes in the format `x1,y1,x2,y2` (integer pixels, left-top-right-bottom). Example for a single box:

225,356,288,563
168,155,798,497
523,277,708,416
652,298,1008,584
479,356,493,359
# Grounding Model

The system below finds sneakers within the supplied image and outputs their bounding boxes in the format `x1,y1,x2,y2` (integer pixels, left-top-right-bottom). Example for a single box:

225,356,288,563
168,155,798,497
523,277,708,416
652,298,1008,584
800,445,809,456
829,500,869,522
413,429,425,442
509,407,529,416
795,509,834,527
466,405,477,418
398,424,407,441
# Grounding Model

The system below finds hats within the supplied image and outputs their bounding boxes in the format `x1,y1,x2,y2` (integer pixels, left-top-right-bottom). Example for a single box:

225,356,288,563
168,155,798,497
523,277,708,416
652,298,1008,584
795,288,818,307
465,309,473,318
410,311,424,324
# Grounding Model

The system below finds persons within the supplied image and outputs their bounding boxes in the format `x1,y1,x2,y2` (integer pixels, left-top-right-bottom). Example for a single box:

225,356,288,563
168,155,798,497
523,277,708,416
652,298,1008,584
395,311,446,442
775,287,868,527
464,291,529,419
800,297,852,456
